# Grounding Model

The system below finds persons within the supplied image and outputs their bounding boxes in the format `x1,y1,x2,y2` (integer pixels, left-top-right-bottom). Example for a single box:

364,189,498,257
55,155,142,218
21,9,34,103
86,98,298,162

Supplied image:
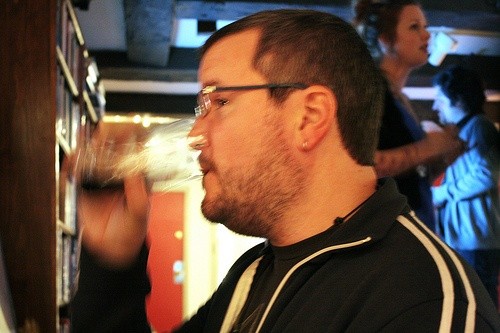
68,1,500,333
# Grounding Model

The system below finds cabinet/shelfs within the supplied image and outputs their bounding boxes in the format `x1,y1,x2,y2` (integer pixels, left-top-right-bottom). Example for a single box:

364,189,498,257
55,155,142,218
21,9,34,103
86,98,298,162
0,0,106,333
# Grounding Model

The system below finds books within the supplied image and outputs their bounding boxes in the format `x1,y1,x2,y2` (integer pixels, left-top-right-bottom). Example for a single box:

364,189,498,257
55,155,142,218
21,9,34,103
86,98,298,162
55,2,101,302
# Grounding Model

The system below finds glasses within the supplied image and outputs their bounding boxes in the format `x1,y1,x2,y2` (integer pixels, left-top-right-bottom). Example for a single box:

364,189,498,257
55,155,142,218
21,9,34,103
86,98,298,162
196,83,309,122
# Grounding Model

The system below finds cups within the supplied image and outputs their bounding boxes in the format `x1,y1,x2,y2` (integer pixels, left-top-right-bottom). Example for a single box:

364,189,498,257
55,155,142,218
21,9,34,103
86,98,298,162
68,120,204,194
